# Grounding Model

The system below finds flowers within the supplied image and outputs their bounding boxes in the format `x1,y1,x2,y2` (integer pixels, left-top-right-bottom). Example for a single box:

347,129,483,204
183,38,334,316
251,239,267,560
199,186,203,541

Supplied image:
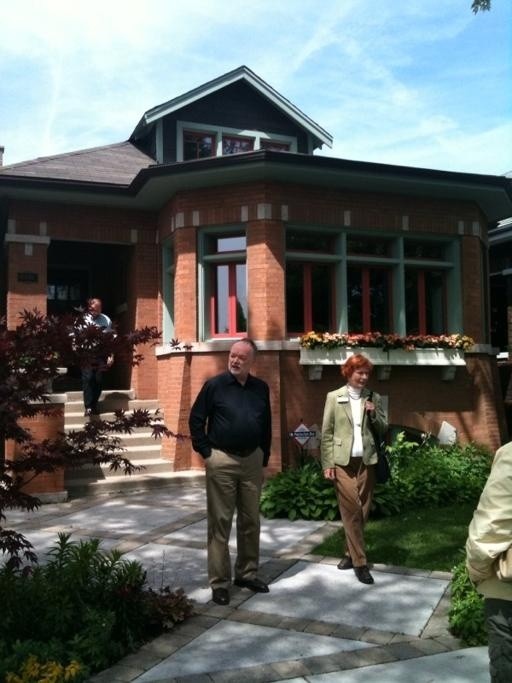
298,329,475,361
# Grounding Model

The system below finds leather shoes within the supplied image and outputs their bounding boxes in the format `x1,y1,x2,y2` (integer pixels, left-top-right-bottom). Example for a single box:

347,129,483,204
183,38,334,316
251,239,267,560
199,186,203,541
338,555,352,568
85,407,93,415
234,577,269,592
355,565,374,583
213,587,229,604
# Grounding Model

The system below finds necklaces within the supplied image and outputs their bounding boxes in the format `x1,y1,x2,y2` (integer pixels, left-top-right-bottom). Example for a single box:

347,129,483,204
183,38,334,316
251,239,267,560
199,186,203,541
348,392,361,401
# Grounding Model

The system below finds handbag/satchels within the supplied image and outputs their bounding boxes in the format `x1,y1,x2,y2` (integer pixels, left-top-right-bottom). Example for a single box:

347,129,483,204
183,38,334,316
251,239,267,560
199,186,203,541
374,453,390,483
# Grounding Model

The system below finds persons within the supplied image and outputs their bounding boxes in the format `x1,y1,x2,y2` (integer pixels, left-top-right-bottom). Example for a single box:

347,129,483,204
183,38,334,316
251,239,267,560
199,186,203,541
189,338,274,605
462,440,511,683
69,296,117,417
317,354,390,584
